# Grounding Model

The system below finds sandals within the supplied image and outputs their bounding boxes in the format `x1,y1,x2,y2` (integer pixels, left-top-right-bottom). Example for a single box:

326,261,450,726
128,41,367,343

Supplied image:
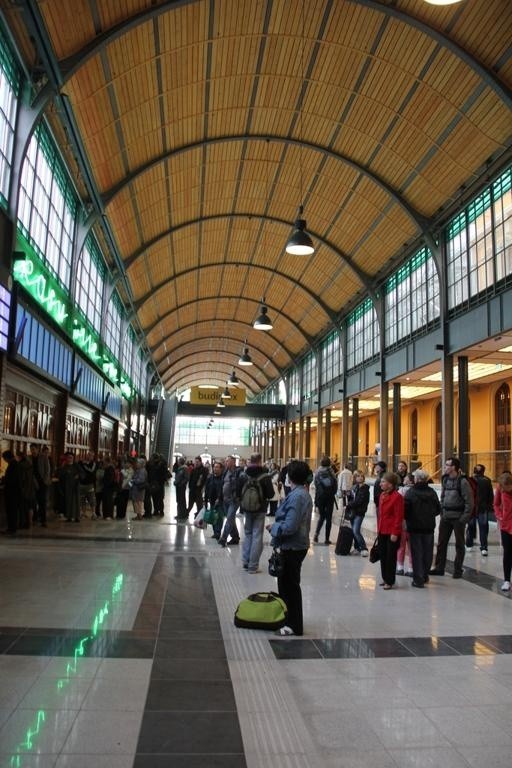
277,626,295,636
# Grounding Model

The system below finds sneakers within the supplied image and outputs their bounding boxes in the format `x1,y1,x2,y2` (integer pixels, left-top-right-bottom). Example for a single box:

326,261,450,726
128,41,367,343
242,563,262,574
501,581,511,590
211,533,240,545
313,534,332,544
481,550,488,555
131,511,165,520
380,569,461,590
352,547,369,557
464,547,473,552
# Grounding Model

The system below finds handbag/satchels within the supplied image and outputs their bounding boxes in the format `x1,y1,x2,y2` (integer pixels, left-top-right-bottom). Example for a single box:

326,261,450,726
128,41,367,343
193,504,219,529
234,592,287,631
268,554,287,576
370,538,380,563
139,481,148,489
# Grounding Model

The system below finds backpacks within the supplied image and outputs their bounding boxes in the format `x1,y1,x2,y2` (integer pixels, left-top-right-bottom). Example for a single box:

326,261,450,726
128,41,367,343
458,475,477,519
240,473,271,512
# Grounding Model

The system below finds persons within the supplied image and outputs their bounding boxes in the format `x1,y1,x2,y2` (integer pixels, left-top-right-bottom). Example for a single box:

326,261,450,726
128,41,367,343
266,460,313,635
0,442,292,574
316,456,512,589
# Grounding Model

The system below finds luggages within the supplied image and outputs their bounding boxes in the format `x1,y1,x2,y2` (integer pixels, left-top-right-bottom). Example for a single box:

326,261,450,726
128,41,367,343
335,506,353,555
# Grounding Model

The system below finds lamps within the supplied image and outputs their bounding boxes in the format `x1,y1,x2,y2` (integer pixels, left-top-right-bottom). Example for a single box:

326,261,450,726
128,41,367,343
282,0,316,260
221,380,231,398
227,364,240,386
237,333,254,366
252,191,274,332
